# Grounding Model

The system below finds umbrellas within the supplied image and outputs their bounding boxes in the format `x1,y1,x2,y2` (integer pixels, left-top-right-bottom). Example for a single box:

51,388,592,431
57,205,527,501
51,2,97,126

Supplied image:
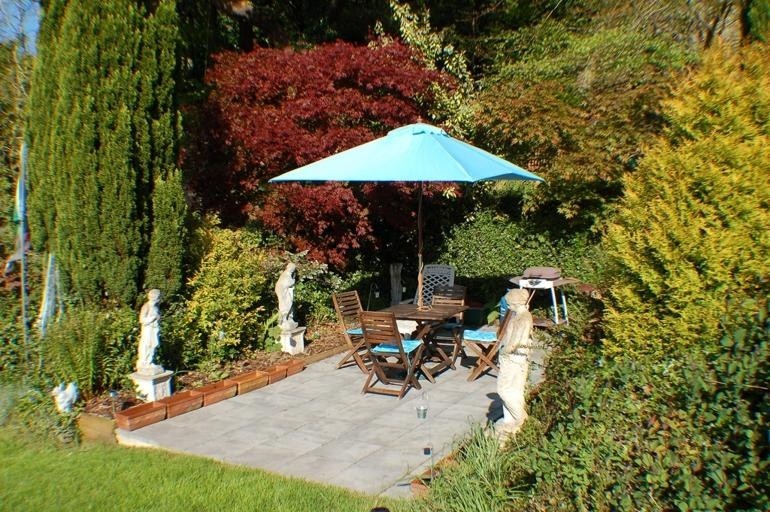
266,114,546,307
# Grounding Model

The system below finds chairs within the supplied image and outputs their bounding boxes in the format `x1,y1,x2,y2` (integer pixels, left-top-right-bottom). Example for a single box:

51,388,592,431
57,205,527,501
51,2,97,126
333,264,512,400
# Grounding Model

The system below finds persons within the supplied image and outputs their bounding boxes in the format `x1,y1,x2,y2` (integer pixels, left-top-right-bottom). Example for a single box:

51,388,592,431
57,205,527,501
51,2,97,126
274,262,296,323
496,288,534,425
137,288,162,367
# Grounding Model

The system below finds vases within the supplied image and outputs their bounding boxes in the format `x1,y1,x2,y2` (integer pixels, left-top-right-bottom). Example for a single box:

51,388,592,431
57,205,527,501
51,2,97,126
114,360,305,431
